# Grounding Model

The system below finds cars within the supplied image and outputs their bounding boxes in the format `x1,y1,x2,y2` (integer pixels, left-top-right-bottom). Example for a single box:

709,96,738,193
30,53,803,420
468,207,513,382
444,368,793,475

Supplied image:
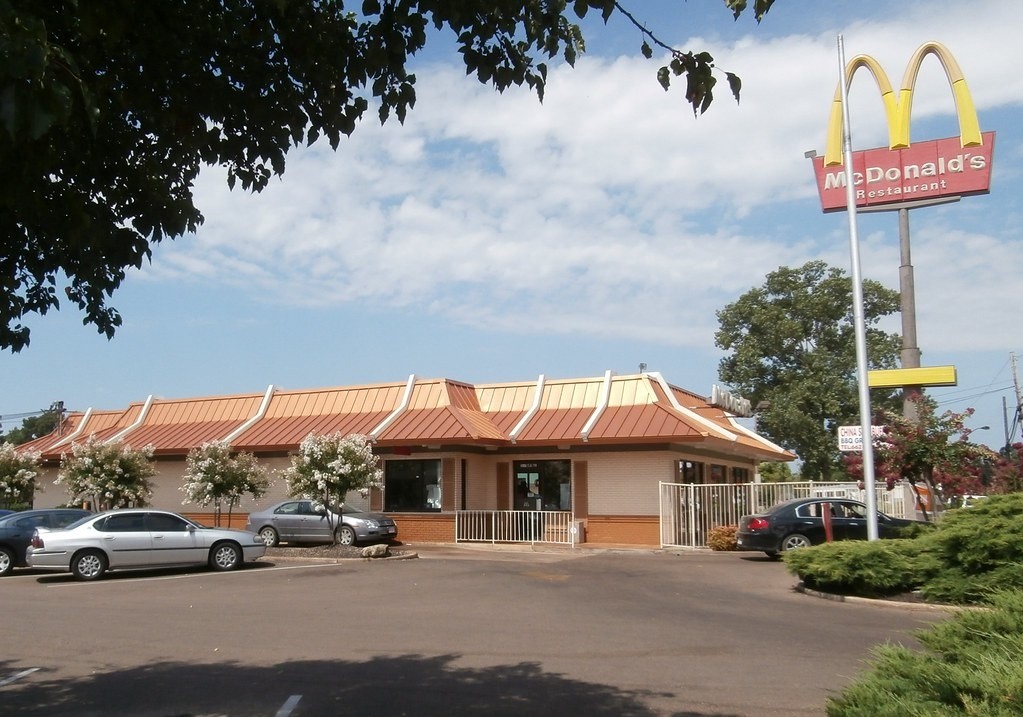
0,508,99,579
26,507,268,583
246,498,398,547
736,496,939,560
